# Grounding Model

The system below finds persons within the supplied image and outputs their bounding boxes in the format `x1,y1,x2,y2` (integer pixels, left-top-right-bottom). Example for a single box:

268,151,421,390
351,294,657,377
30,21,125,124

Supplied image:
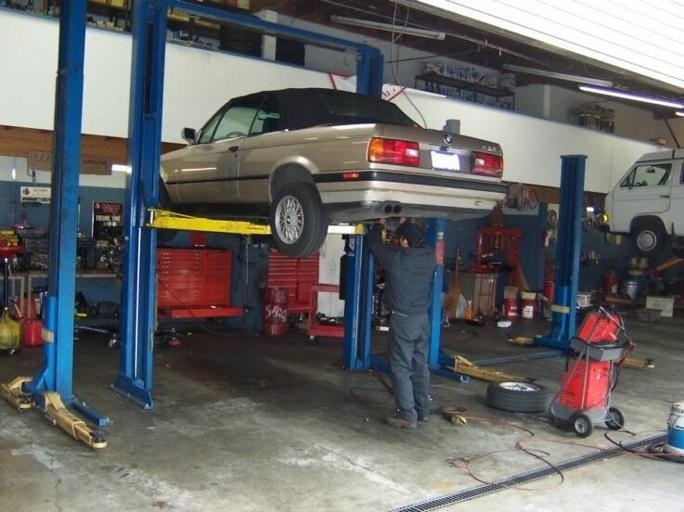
364,216,437,427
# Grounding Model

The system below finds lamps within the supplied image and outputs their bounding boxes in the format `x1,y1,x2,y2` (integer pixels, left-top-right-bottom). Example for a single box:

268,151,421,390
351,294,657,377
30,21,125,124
327,13,446,40
501,63,683,117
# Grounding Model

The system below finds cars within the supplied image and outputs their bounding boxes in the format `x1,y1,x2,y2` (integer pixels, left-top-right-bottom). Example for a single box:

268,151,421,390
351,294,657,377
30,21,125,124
158,87,508,259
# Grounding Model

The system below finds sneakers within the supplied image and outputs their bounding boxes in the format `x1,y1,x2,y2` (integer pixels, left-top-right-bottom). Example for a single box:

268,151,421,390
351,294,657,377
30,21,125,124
385,416,417,429
420,416,429,421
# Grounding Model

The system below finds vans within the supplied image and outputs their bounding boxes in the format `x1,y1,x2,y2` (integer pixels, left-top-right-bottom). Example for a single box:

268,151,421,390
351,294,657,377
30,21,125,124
595,148,684,255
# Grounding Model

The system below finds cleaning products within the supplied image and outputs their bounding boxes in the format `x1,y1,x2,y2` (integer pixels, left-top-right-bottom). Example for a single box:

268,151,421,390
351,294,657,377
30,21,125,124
463,297,477,319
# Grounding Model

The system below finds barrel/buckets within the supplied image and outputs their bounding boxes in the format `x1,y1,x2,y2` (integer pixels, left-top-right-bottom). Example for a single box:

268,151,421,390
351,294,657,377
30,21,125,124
626,279,640,299
543,280,555,297
577,292,593,308
1,305,22,351
502,299,519,318
604,276,619,293
522,298,540,319
665,400,684,455
20,316,46,346
